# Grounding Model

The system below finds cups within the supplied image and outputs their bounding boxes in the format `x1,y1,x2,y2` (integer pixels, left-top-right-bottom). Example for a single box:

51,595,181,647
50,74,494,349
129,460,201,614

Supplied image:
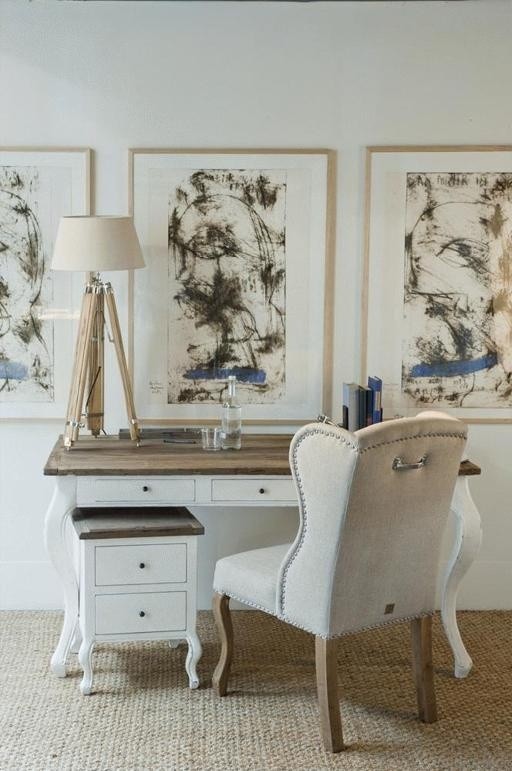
201,427,223,451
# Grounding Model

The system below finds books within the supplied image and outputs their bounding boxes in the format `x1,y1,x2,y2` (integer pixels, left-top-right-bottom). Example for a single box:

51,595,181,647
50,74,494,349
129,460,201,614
342,376,382,432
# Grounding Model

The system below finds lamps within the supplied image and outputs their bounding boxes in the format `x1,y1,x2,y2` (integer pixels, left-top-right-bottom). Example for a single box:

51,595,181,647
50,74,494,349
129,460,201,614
49,213,150,453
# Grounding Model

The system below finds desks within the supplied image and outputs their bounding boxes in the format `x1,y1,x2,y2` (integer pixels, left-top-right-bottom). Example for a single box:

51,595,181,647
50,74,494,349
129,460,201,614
42,433,482,680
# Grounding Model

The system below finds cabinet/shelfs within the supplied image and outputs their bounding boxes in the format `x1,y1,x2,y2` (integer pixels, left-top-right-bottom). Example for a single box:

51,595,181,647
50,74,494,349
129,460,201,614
73,534,204,696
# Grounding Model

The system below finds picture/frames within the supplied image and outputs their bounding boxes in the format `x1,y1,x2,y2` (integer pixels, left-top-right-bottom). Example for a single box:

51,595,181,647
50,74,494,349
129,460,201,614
360,144,512,424
0,144,93,425
127,147,339,426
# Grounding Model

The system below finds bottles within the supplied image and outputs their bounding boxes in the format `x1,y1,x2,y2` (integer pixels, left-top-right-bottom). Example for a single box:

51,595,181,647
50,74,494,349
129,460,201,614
222,375,242,450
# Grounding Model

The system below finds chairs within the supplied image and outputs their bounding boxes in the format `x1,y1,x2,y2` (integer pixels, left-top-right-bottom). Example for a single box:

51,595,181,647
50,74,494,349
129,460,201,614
203,409,468,754
